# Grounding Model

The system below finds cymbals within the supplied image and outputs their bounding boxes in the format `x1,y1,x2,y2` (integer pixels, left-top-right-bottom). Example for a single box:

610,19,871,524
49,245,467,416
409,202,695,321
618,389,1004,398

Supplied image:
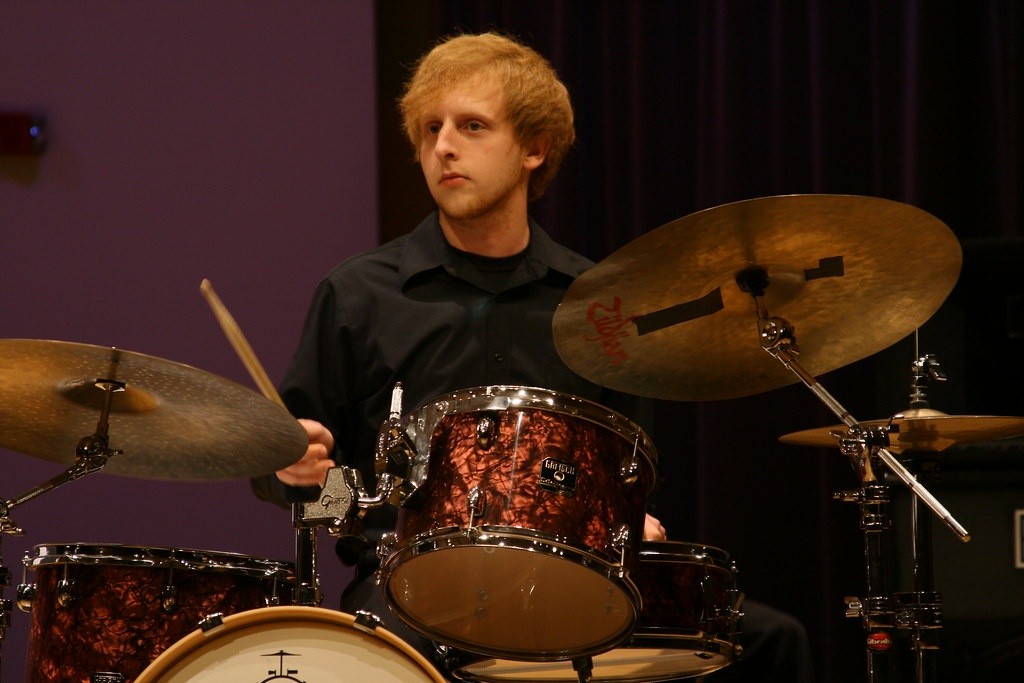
549,193,962,401
776,407,1024,453
1,337,310,484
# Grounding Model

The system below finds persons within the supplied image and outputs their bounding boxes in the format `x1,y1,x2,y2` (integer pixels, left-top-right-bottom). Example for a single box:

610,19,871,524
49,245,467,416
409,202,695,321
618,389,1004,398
252,29,813,683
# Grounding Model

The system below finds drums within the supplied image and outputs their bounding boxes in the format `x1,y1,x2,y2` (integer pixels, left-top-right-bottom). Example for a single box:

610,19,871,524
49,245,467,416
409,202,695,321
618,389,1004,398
379,383,658,663
19,541,305,683
124,606,452,683
444,540,744,681
